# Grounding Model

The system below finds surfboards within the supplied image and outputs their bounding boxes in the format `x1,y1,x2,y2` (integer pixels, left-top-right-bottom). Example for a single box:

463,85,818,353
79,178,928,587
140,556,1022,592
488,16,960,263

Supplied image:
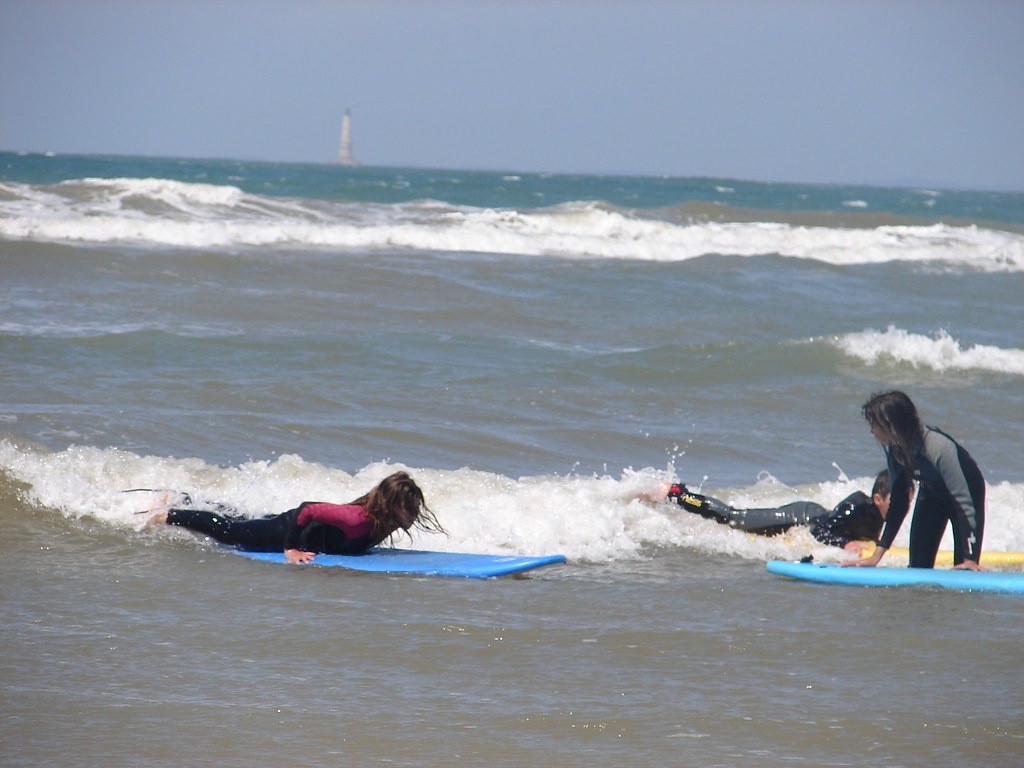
207,547,568,580
765,556,1024,595
747,533,1023,569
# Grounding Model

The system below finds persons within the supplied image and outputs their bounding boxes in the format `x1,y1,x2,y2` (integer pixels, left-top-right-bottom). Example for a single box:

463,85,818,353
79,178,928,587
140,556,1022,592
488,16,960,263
167,471,442,564
665,469,914,558
840,391,989,571
